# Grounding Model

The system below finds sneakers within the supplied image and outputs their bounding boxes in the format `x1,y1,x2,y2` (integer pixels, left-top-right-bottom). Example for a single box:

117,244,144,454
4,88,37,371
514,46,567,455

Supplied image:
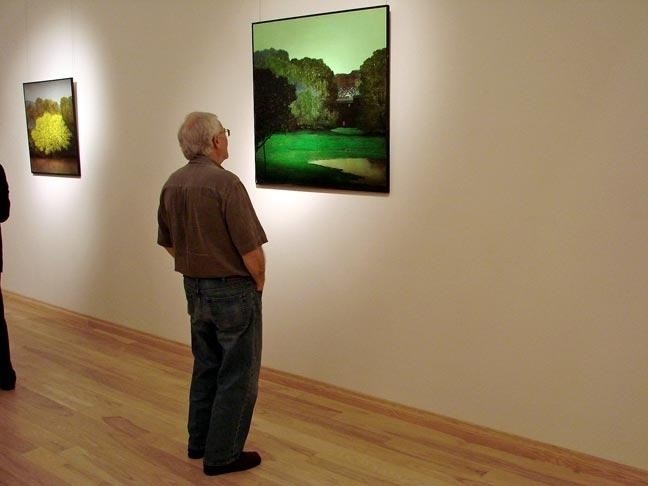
187,450,261,476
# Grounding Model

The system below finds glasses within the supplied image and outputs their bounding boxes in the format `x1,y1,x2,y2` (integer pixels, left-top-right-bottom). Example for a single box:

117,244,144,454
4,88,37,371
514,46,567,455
212,129,230,144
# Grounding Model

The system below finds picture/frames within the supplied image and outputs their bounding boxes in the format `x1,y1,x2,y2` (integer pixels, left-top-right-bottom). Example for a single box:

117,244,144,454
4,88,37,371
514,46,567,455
21,76,81,177
248,1,390,193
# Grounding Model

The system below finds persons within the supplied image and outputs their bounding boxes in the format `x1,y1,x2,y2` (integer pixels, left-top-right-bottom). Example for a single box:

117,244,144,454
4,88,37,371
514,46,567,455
0,160,17,391
156,113,268,475
